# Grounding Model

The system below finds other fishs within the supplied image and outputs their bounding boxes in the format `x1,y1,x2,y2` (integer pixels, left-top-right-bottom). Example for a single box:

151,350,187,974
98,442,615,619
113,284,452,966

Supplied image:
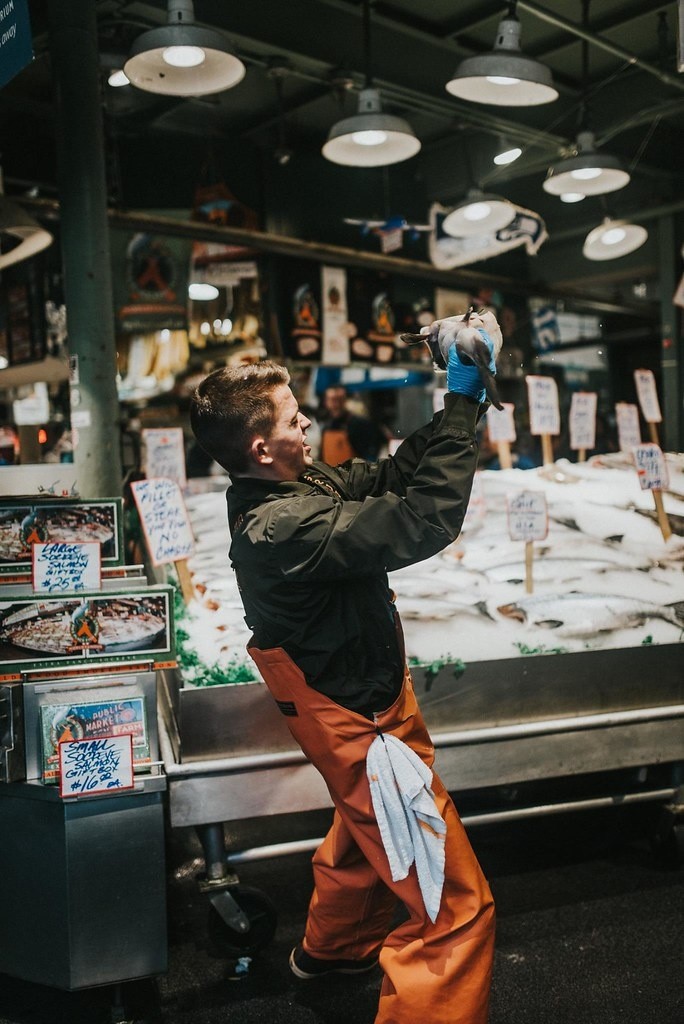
183,490,250,664
400,306,505,411
390,451,683,634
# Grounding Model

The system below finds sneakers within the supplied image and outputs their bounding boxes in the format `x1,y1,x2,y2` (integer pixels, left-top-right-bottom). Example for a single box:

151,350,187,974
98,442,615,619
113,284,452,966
289,944,380,980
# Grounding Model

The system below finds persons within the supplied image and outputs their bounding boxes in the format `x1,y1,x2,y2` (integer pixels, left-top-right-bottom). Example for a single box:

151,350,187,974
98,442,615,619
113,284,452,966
183,306,513,1023
312,383,383,468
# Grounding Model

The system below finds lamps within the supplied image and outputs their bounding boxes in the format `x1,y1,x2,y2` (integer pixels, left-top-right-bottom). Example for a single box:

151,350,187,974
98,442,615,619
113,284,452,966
583,213,649,261
542,132,631,198
321,0,422,168
121,0,246,100
441,132,516,238
108,70,131,88
443,1,558,107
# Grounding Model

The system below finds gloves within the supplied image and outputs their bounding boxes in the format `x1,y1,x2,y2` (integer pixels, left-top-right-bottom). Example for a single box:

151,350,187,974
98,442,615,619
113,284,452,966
478,360,497,405
446,328,495,401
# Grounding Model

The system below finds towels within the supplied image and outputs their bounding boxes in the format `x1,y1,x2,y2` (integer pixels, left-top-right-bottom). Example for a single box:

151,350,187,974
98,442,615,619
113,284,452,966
366,730,449,925
